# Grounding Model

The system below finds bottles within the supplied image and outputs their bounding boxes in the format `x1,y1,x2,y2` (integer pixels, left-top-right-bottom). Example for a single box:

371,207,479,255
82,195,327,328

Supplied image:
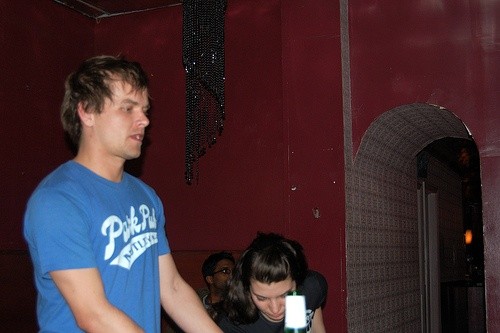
283,291,307,333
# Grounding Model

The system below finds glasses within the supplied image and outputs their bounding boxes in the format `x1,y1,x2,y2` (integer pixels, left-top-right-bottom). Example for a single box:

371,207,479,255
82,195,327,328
213,268,231,274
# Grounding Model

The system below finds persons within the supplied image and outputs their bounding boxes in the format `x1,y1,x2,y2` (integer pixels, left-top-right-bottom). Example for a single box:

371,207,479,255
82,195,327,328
166,250,236,333
215,230,328,333
23,52,225,333
